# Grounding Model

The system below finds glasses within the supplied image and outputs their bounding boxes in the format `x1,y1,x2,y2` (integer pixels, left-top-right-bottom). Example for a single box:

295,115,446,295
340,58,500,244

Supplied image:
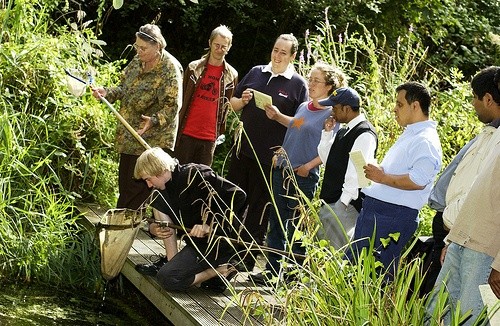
309,79,325,85
133,42,148,52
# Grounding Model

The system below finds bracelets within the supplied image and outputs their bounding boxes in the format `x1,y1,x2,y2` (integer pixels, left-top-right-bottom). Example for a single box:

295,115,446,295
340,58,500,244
152,117,157,126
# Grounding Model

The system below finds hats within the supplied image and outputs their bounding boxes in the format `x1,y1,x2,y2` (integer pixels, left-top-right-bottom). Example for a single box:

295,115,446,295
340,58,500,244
318,87,360,107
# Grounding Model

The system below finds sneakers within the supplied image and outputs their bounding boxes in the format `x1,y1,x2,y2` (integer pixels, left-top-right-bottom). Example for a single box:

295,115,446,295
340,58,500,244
274,280,295,289
248,273,274,286
210,265,238,293
135,253,168,277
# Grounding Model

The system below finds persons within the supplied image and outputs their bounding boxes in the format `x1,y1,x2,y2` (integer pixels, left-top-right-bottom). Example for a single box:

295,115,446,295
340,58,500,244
425,67,500,326
92,23,379,291
343,81,443,293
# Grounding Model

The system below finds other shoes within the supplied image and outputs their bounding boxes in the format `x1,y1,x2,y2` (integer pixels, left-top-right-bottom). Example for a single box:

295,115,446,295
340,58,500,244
229,259,251,271
